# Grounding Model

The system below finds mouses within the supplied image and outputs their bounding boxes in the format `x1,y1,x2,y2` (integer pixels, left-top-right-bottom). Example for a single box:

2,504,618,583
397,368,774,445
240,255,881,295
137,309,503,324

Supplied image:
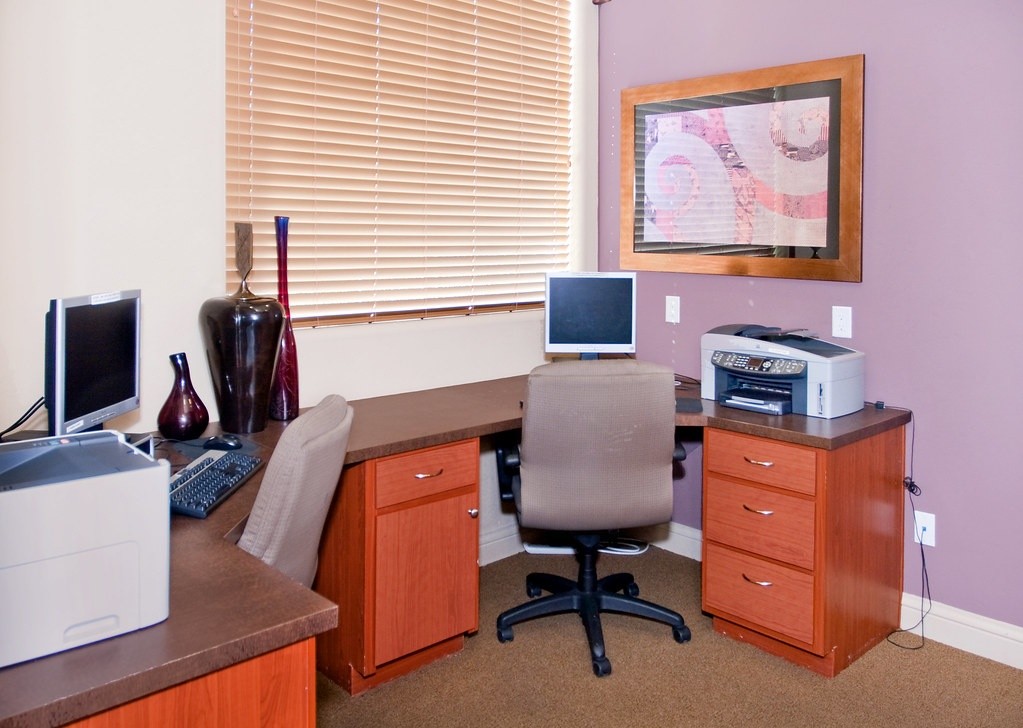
203,434,242,451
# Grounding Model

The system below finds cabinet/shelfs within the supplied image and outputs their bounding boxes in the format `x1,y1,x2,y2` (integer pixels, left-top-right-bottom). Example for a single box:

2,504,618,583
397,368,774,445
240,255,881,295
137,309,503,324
316,438,479,697
702,418,906,679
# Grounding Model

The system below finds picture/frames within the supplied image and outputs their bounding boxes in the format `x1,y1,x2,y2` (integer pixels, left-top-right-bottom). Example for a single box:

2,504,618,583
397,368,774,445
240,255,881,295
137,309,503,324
620,55,868,283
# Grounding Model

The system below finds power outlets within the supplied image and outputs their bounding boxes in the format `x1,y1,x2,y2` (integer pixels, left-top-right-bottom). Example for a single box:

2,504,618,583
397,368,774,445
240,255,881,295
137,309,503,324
666,295,680,323
913,509,935,547
832,306,852,338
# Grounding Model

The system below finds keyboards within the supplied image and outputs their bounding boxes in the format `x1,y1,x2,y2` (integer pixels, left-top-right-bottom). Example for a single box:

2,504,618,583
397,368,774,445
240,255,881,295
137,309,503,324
169,450,266,519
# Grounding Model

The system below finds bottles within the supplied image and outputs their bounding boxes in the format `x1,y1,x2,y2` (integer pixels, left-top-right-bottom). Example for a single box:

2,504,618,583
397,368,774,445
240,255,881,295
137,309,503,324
198,281,285,435
269,216,300,421
157,353,209,443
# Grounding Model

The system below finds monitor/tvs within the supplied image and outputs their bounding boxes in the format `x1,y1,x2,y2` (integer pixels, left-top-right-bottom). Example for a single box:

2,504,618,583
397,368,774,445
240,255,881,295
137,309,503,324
544,271,637,361
43,289,140,436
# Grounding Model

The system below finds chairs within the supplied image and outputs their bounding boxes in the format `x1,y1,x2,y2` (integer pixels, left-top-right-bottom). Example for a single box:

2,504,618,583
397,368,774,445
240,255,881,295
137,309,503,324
236,393,351,593
496,360,692,676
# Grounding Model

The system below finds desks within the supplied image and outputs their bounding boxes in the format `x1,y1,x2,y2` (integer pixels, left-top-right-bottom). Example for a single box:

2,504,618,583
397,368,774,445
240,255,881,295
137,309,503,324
1,362,911,728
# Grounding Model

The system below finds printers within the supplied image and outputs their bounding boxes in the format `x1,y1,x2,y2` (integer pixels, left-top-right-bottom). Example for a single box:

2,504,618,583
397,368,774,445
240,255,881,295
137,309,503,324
0,429,172,669
700,324,864,420
